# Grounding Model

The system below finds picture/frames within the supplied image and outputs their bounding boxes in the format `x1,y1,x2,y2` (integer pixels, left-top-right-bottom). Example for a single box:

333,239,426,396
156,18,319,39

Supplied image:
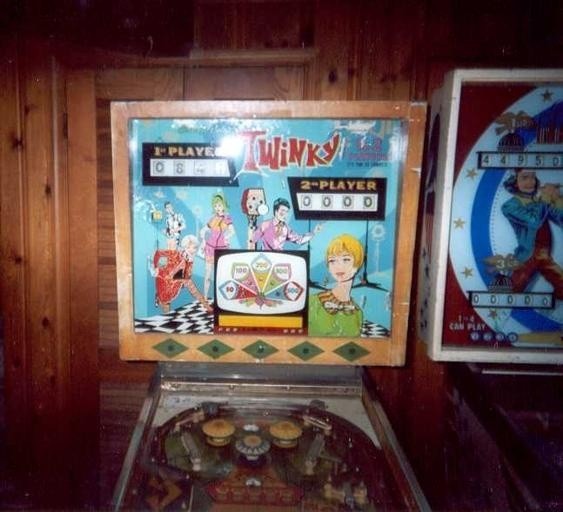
107,88,429,368
428,65,562,367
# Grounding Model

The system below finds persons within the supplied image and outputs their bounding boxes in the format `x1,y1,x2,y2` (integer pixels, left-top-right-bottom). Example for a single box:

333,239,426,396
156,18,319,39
501,168,562,300
133,195,363,337
